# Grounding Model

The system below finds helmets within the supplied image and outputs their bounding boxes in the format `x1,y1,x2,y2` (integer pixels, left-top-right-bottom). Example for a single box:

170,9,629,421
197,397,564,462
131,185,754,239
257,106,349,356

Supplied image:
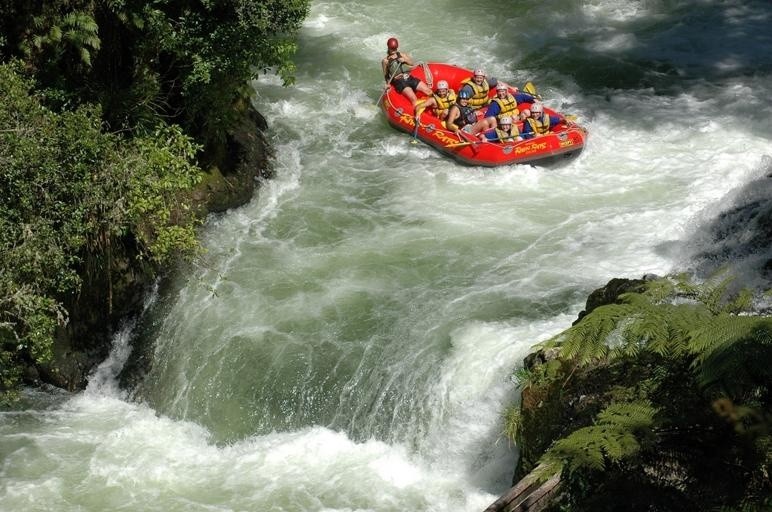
458,90,470,99
437,80,448,92
388,38,398,49
496,82,508,90
500,117,512,130
530,103,543,118
474,69,485,77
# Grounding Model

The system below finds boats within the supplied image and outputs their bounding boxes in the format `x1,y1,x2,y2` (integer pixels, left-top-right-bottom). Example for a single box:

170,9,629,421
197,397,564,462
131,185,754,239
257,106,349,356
384,64,589,168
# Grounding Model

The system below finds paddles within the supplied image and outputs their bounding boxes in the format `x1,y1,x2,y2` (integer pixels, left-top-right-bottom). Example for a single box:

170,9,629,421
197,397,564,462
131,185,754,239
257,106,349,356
522,81,535,96
409,100,426,144
443,131,535,148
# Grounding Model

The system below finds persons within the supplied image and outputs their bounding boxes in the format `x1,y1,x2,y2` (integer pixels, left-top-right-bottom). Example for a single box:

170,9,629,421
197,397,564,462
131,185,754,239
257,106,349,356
446,90,497,135
524,101,571,139
381,38,434,110
461,68,520,109
413,80,457,124
484,82,539,120
479,117,524,144
